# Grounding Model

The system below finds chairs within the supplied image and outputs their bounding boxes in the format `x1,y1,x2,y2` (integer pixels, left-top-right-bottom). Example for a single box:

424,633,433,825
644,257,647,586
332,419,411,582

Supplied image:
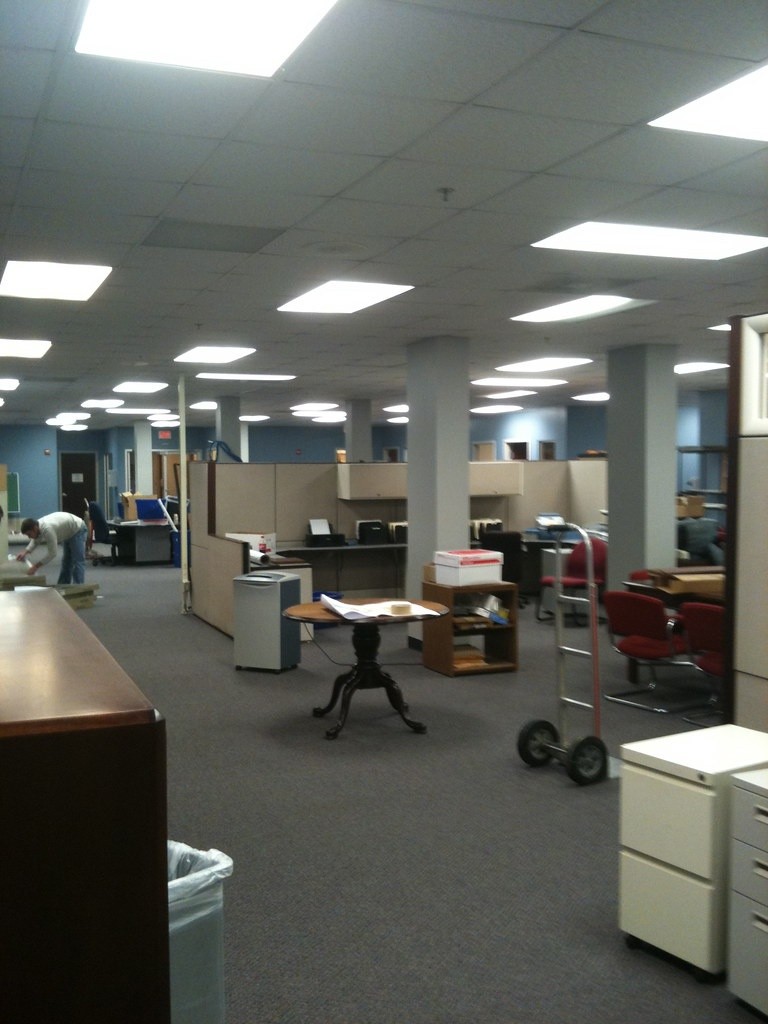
88,503,120,567
534,538,607,626
604,592,709,712
479,531,528,609
681,603,729,726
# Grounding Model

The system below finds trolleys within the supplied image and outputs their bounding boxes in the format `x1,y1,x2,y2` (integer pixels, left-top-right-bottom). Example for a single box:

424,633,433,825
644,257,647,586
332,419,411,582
516,521,613,786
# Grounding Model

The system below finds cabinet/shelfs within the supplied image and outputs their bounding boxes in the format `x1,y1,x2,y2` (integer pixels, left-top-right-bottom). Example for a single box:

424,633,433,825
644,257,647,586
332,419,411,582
0,586,167,1024
617,724,768,981
423,580,520,676
729,769,768,1017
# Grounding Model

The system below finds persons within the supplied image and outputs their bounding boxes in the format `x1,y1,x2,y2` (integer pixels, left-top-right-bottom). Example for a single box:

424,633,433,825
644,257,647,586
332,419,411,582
15,511,90,584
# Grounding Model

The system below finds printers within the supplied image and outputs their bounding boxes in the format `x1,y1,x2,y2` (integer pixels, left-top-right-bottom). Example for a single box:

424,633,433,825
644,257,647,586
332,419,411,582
305,523,344,548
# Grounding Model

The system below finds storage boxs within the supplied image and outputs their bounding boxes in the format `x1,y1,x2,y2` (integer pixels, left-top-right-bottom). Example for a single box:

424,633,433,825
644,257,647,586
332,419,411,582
136,498,167,520
676,494,703,519
435,549,502,585
120,492,158,521
171,530,191,568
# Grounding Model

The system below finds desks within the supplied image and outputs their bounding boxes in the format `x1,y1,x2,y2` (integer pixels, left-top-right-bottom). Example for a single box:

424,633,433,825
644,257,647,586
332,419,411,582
250,538,583,644
107,520,180,563
282,598,451,738
0,557,45,584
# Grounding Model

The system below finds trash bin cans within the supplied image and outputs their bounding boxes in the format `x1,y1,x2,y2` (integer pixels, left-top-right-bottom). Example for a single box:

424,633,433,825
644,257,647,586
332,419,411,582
168,838,235,1024
